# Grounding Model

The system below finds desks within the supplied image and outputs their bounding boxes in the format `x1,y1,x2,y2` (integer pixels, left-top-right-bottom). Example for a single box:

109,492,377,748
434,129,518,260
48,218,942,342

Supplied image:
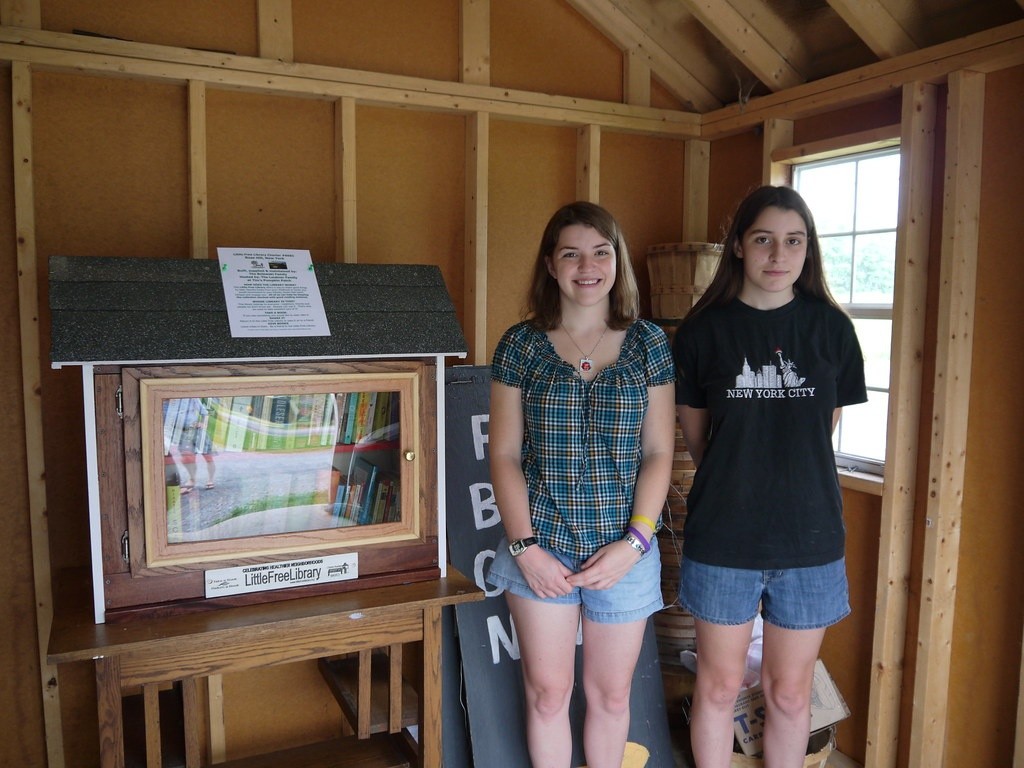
45,562,486,768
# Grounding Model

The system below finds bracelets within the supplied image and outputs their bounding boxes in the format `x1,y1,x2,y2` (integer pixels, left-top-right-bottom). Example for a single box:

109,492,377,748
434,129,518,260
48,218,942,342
624,534,645,557
625,526,650,552
630,514,656,530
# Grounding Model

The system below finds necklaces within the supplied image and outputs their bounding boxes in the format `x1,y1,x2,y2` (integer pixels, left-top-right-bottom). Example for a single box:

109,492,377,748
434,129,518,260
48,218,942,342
560,321,609,373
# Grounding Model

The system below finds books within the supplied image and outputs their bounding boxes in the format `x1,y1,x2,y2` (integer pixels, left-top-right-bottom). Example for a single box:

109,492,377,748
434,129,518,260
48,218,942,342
657,419,697,679
161,391,400,456
166,457,401,544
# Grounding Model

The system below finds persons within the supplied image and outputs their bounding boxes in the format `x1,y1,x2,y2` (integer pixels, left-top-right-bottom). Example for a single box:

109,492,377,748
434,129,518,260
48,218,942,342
673,186,868,768
484,200,676,768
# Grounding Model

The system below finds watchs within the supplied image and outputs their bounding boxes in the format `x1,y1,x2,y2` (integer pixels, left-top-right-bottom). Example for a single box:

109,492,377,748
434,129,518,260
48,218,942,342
508,536,537,557
